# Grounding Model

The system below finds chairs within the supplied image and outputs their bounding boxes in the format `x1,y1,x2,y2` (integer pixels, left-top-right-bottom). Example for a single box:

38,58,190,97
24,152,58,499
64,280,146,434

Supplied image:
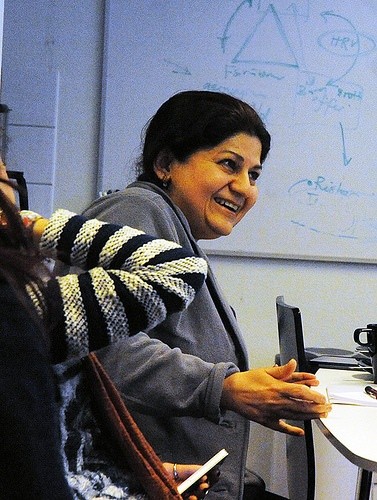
274,294,318,500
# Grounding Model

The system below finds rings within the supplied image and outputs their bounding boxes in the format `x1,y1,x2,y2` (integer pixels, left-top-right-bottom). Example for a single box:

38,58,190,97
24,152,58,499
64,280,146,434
172,463,178,478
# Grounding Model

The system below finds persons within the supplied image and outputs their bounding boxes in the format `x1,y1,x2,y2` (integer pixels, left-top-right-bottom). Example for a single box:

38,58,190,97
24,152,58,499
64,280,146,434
0,163,208,500
52,90,332,500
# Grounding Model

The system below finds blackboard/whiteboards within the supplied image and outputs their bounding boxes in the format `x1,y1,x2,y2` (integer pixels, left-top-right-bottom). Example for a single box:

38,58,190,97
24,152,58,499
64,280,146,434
97,1,377,264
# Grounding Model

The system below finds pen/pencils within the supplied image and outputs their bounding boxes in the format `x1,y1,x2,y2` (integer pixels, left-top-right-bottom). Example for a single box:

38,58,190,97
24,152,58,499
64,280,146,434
363,385,377,400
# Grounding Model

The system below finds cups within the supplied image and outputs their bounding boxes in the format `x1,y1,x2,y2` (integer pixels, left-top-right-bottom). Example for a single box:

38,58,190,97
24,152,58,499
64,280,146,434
354,323,377,384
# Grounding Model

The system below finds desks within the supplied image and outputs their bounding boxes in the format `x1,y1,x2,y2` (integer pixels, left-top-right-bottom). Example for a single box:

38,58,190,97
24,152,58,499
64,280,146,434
274,346,376,500
